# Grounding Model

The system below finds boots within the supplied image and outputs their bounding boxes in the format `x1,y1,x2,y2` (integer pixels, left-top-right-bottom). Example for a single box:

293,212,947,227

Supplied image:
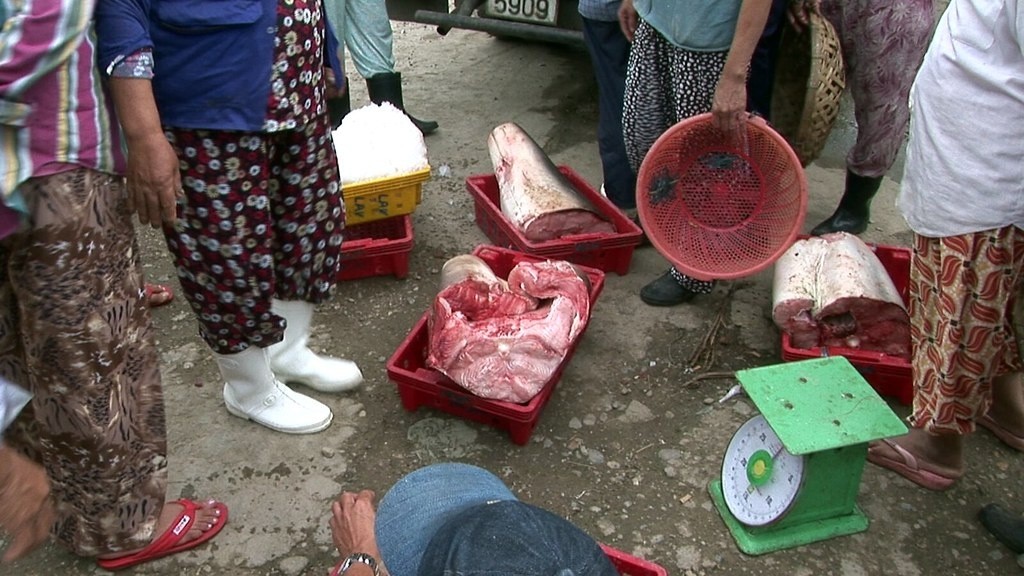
265,298,363,391
367,72,438,133
810,166,884,236
218,346,334,434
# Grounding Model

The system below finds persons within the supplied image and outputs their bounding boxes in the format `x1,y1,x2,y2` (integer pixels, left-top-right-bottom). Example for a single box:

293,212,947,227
0,0,363,576
579,0,936,307
329,461,619,576
866,0,1024,492
325,0,438,141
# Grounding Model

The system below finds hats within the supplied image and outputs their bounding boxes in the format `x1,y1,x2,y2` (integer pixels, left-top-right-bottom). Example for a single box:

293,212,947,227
375,463,619,576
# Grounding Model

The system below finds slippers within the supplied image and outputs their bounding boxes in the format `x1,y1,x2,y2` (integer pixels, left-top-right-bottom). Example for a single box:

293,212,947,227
867,438,955,491
976,414,1024,450
144,283,175,306
97,500,227,568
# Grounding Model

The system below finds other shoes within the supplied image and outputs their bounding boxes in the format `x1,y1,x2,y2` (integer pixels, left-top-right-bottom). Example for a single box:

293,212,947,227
641,265,716,306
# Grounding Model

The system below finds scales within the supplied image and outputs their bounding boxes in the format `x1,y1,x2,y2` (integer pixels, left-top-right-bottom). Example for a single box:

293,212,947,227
707,354,910,557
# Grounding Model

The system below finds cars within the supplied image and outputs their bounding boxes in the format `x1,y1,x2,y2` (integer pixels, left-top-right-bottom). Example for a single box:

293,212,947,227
384,1,588,52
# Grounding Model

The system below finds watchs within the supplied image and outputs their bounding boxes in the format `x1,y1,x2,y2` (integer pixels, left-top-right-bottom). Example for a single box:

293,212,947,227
337,552,379,576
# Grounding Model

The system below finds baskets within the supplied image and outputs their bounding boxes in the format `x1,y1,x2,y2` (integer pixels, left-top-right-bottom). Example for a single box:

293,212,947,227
635,108,807,280
767,11,846,168
